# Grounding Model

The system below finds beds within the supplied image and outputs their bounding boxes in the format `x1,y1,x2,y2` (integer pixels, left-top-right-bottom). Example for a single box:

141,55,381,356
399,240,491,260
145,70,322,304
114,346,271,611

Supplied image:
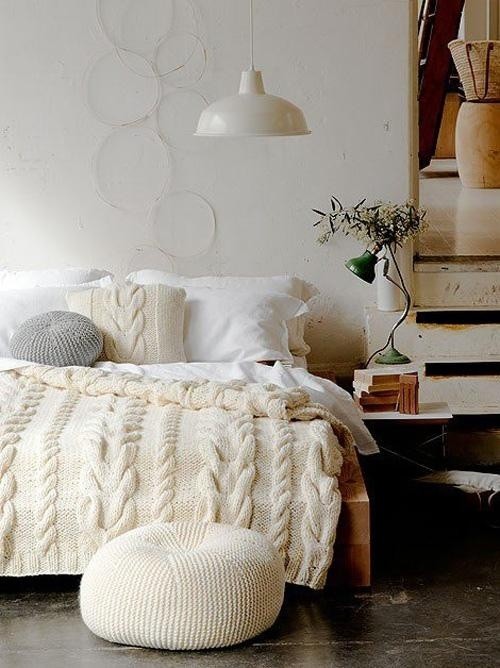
1,350,374,589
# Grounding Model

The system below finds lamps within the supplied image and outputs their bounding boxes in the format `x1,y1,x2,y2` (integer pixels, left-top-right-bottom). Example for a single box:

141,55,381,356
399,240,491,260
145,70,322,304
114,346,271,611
194,1,311,139
342,241,412,365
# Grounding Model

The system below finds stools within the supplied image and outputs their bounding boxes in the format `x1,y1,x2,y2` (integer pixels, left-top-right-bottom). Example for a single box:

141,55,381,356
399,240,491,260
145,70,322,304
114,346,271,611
79,522,284,650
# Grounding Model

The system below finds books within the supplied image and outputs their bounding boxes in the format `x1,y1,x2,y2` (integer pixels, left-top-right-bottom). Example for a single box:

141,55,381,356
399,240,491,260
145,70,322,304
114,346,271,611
352,368,418,413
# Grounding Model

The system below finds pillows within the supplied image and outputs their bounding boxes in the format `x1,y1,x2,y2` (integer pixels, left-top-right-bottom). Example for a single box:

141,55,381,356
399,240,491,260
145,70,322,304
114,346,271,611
67,285,187,365
3,269,115,349
125,269,313,357
183,286,308,365
9,311,103,368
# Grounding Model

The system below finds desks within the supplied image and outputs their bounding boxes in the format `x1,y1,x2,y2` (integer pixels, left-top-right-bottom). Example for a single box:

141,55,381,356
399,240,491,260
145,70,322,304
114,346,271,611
359,400,454,473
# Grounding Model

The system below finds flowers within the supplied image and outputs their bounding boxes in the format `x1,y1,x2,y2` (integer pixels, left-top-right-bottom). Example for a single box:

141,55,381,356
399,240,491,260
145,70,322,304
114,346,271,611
308,193,428,254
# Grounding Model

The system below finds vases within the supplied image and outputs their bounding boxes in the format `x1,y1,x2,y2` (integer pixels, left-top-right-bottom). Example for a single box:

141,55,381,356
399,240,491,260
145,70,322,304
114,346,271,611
454,101,500,190
377,242,402,312
